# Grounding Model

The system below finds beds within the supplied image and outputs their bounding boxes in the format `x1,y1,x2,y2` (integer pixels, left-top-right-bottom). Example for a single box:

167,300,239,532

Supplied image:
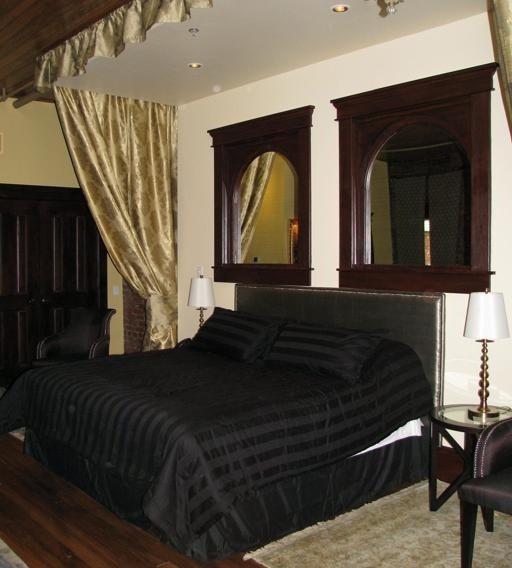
27,286,447,554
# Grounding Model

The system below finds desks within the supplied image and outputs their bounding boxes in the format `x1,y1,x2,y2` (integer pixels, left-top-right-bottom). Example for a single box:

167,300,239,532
425,403,511,511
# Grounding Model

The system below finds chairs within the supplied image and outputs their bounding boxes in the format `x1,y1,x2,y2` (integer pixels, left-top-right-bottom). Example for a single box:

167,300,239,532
26,305,116,373
456,417,512,568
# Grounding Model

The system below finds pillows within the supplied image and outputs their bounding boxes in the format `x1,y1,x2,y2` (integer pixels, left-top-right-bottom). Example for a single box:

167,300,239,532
189,303,392,387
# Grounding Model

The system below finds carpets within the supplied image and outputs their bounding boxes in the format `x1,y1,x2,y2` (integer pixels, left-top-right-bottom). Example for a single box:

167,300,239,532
242,476,512,568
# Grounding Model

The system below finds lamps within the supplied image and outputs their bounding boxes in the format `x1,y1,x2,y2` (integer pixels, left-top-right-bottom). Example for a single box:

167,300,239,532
462,286,511,417
186,266,216,330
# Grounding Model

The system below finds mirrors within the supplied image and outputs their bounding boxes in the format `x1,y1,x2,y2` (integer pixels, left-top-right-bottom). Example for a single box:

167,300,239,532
330,60,499,291
206,105,316,284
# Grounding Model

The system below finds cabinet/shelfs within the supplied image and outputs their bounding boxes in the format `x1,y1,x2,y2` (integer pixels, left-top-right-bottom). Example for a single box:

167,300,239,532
2,180,109,382
